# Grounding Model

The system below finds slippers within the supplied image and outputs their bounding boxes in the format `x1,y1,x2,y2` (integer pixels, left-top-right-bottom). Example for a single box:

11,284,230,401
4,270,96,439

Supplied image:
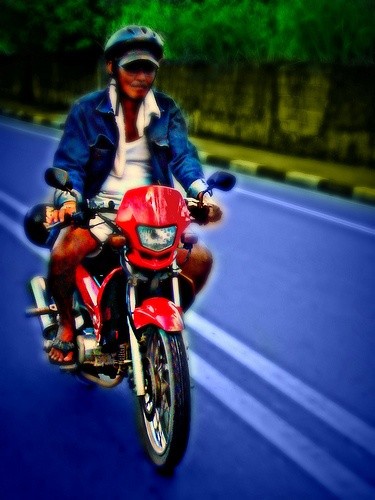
48,336,75,365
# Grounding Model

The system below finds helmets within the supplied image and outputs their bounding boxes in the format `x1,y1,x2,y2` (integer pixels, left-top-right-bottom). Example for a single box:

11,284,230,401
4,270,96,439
103,24,164,67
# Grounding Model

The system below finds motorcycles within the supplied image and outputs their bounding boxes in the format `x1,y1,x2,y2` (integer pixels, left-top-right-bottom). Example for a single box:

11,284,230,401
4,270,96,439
23,162,236,477
